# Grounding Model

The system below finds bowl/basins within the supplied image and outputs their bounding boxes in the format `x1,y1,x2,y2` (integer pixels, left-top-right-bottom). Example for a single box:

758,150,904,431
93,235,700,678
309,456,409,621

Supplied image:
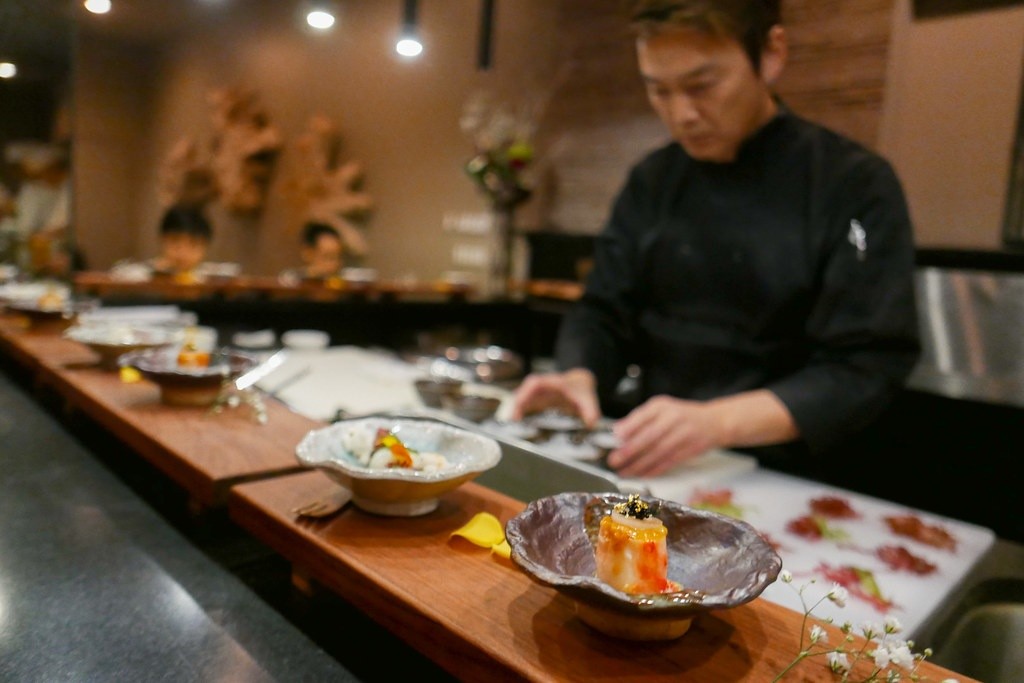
505,492,783,642
295,416,502,517
118,347,258,407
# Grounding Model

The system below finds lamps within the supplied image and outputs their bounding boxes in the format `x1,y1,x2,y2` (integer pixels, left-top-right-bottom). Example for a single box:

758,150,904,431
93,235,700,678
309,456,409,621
397,0,424,58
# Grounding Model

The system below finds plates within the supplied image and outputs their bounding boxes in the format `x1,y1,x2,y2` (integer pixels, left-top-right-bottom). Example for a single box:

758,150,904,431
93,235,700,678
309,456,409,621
62,308,213,370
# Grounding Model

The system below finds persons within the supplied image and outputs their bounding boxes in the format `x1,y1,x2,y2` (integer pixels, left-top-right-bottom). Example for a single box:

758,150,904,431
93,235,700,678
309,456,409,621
280,224,376,289
494,0,923,492
112,203,243,283
0,138,89,275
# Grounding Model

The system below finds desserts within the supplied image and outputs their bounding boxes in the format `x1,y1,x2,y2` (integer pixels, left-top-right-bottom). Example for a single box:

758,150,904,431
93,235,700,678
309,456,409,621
594,493,668,595
171,326,218,367
360,426,423,471
